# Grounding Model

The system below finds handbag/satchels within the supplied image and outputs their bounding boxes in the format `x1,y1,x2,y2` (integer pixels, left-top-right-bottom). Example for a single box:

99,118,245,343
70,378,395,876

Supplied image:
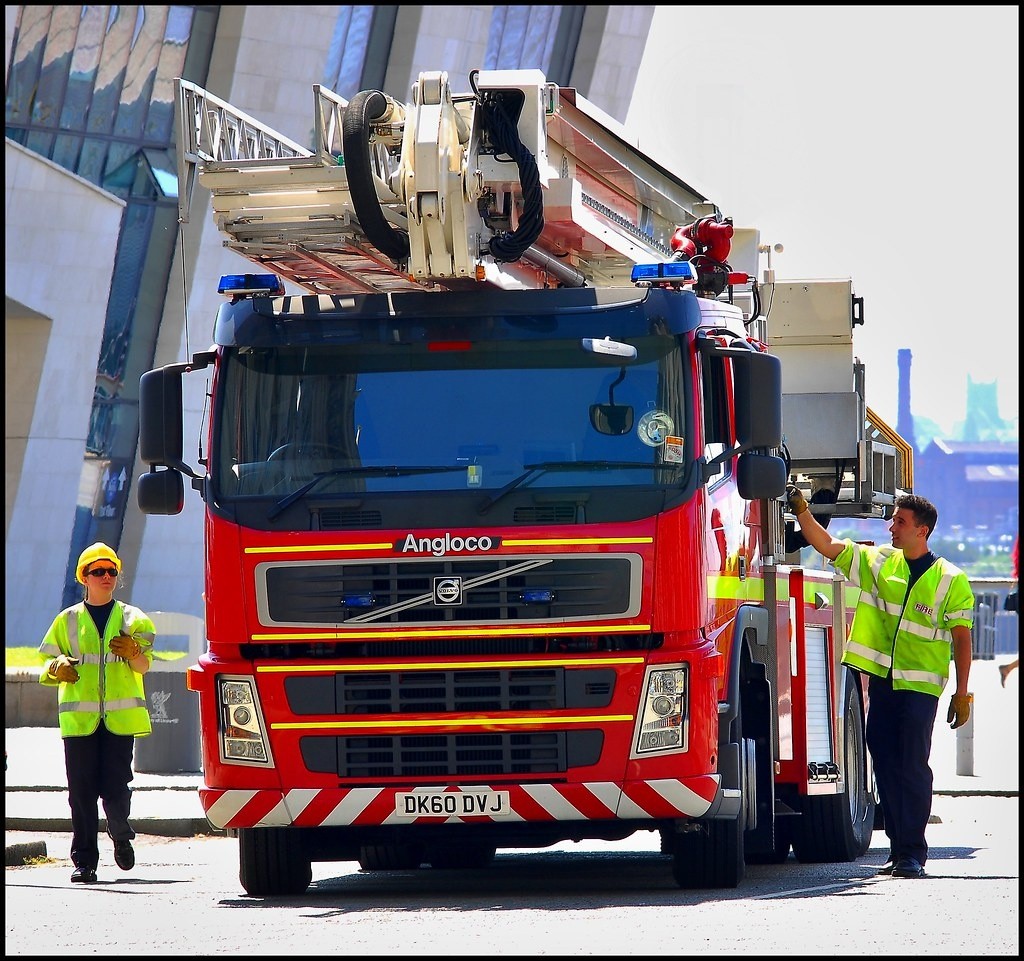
1004,578,1018,611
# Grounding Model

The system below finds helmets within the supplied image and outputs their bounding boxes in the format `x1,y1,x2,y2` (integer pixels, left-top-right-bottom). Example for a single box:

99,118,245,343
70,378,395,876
76,542,121,584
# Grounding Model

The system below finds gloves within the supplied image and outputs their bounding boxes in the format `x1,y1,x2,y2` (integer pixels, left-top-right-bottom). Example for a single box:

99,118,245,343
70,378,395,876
110,630,140,660
786,484,808,515
49,655,79,684
947,694,972,729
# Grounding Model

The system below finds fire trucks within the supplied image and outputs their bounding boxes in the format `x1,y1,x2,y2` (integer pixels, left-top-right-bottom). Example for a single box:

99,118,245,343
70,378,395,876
135,64,919,898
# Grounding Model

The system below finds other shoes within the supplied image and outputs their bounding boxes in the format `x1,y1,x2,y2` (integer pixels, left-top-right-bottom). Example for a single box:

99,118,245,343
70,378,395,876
892,856,925,877
106,826,135,870
878,860,892,874
999,665,1009,688
71,866,97,882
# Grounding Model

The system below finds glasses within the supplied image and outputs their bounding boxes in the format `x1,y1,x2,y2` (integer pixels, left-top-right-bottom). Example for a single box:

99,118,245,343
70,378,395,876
84,568,118,577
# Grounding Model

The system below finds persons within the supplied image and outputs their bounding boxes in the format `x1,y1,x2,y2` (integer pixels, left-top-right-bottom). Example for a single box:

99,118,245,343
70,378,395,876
39,541,156,883
786,485,975,876
998,659,1019,688
